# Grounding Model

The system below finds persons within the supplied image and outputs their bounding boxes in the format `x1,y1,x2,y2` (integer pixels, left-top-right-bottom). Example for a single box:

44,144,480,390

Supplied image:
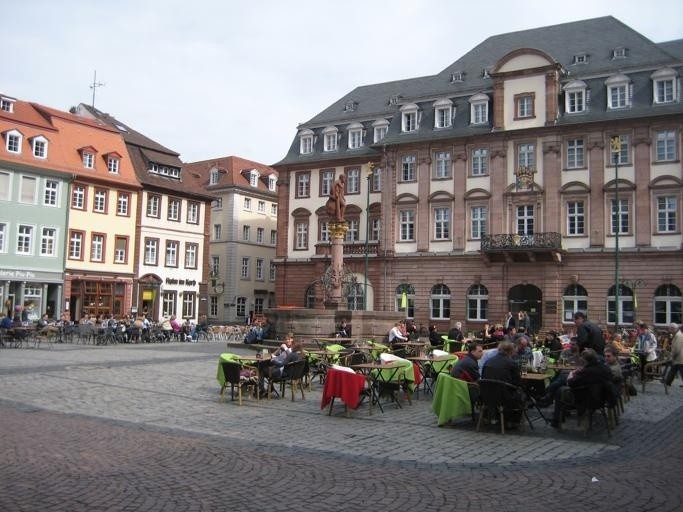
660,324,682,387
257,332,304,398
448,322,465,352
337,318,351,345
428,324,444,345
330,173,347,222
388,321,416,348
452,309,657,429
0,298,272,343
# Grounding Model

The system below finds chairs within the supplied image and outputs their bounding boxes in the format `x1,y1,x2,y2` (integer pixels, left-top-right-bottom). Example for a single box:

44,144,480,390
1,313,271,351
214,315,683,439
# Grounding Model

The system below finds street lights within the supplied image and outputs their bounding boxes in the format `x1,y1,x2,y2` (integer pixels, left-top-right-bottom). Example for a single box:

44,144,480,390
609,136,625,330
361,161,375,309
